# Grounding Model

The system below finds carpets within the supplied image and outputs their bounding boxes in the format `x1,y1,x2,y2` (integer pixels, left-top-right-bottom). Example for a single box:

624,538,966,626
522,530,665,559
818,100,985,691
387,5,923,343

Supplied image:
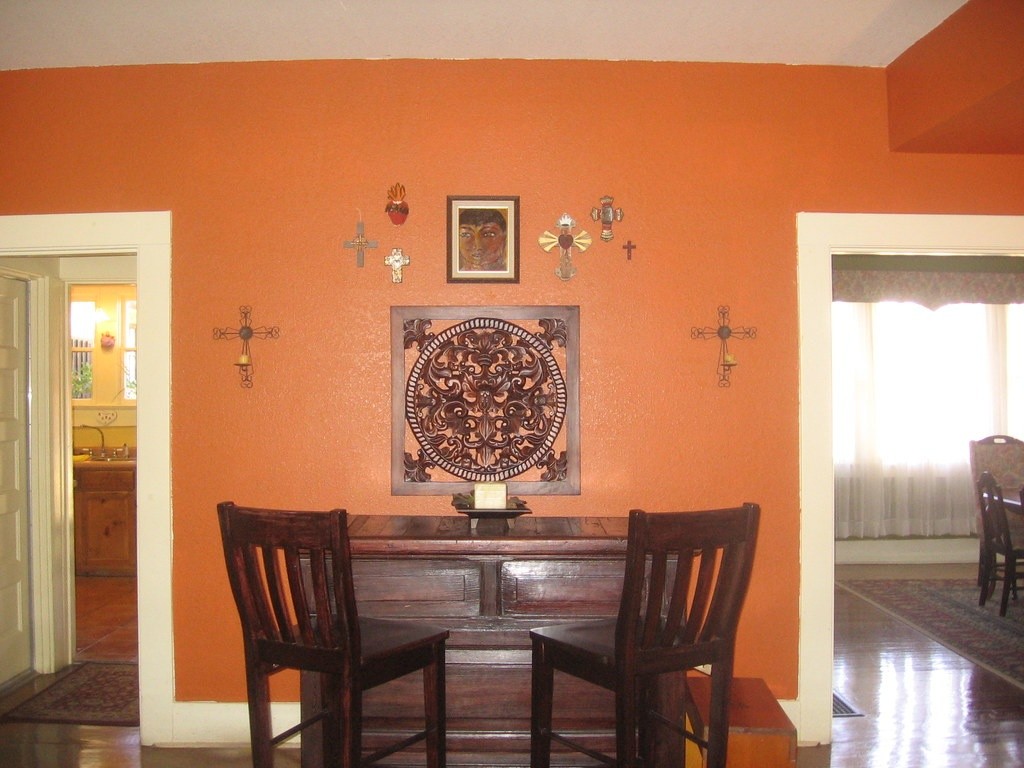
835,578,1024,688
0,660,142,727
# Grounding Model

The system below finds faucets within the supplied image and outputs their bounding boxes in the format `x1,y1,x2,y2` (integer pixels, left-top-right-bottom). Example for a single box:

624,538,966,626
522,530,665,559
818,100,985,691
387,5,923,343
79,423,108,458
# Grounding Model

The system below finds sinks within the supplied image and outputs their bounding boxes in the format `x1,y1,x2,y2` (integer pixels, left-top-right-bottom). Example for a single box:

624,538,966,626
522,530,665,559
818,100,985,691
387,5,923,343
84,455,137,462
73,454,91,463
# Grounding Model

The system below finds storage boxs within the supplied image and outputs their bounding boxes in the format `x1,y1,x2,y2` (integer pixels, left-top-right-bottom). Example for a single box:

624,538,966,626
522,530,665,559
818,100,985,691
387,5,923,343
685,676,798,768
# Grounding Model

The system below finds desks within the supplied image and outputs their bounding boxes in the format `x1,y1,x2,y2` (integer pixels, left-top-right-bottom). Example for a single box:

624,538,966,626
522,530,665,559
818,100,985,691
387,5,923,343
979,487,1024,517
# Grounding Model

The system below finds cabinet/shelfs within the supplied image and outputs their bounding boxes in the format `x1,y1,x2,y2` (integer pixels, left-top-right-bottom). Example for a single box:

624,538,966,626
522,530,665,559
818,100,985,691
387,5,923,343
300,513,703,768
74,469,144,577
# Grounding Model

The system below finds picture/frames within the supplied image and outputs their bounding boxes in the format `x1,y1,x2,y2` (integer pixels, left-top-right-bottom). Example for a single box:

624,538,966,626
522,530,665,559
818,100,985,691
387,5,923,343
446,194,520,284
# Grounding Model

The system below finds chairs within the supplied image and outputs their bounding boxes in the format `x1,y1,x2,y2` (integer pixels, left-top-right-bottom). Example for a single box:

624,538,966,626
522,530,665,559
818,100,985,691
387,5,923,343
967,434,1024,618
527,503,760,768
216,500,450,768
